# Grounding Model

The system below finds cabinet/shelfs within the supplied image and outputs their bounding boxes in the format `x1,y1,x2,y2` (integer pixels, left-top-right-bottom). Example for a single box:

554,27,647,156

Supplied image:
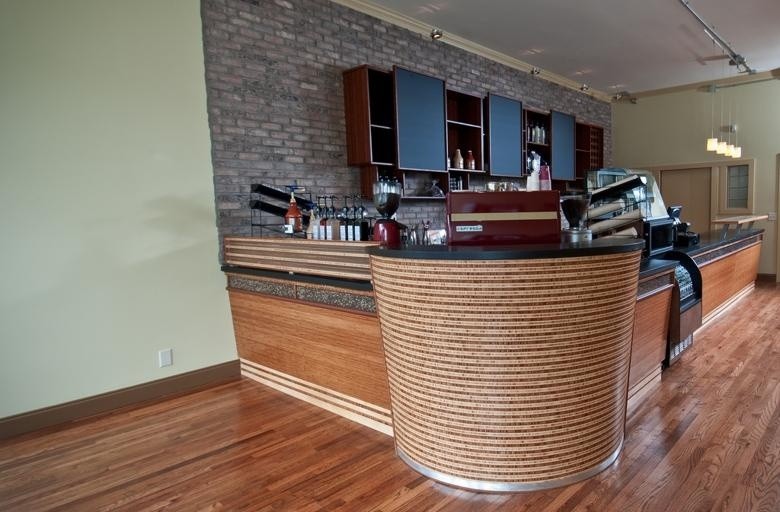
342,64,605,200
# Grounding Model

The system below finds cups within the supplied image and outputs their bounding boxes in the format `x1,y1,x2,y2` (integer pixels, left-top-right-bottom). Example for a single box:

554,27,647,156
526,165,552,191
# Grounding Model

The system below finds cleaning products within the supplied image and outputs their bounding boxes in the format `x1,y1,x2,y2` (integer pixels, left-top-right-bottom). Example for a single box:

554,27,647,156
308,195,367,241
285,184,302,232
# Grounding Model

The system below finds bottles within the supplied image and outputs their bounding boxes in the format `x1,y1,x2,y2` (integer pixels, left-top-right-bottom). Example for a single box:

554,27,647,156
464,150,476,170
527,121,546,144
453,149,464,169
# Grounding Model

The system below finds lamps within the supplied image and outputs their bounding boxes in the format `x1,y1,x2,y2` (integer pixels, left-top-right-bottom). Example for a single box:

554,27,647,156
704,29,743,159
430,29,442,40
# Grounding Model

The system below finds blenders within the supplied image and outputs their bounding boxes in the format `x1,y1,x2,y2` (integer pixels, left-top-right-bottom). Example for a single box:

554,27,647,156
372,177,402,243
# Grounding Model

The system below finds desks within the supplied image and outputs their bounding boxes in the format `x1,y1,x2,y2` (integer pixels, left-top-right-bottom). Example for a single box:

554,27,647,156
711,214,768,231
